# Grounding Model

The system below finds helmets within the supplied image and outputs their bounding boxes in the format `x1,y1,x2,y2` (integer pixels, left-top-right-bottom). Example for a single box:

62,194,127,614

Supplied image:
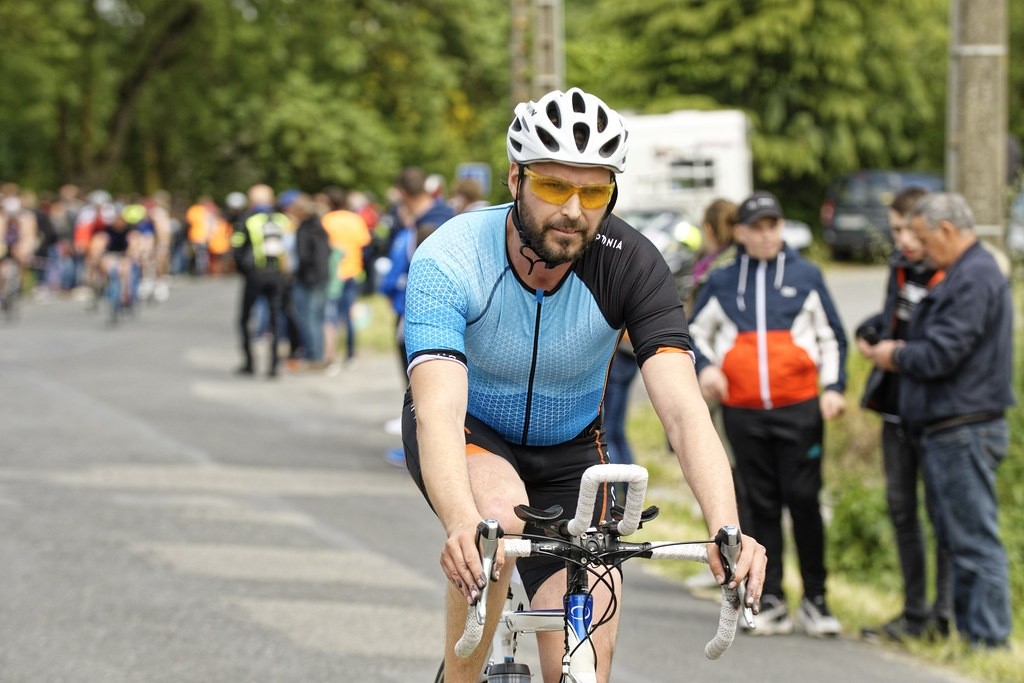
505,87,630,174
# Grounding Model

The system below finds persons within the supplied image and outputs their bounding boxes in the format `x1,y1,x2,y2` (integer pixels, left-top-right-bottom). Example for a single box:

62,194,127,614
0,180,245,317
401,88,767,683
227,167,489,468
853,186,1014,651
687,194,848,637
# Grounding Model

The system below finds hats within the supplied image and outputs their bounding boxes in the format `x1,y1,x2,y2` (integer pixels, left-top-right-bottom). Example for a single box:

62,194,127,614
739,191,784,223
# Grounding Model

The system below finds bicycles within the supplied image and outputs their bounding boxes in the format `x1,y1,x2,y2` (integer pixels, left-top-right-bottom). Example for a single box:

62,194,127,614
435,464,758,683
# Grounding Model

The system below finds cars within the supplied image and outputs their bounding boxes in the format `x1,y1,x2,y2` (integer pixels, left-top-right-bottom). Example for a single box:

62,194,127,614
820,169,945,265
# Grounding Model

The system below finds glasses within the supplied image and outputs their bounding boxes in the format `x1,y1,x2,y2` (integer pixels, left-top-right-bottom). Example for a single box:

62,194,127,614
523,167,615,211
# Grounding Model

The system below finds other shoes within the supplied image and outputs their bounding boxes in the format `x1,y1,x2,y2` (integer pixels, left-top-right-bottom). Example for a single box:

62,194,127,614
860,610,955,648
797,597,841,637
751,609,792,637
